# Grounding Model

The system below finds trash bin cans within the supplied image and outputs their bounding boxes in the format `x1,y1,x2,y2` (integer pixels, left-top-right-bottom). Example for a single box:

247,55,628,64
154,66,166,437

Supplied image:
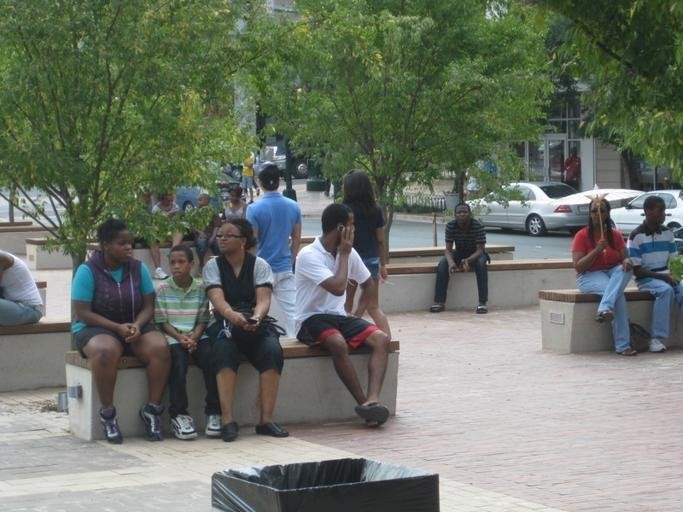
212,458,439,512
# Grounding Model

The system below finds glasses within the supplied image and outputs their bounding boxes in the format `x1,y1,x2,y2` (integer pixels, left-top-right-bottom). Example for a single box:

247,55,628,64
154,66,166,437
216,233,243,238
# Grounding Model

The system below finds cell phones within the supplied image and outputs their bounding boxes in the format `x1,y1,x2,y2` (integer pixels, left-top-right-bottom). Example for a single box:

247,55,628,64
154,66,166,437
337,224,345,232
247,319,257,324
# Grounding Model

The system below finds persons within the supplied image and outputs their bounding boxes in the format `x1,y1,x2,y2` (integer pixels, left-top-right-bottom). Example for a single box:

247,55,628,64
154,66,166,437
0,250,44,327
153,245,223,440
246,164,296,337
294,204,390,428
429,201,490,313
465,153,497,200
342,169,391,341
571,198,636,355
626,196,682,353
138,151,260,279
561,146,581,191
70,219,172,444
201,219,289,442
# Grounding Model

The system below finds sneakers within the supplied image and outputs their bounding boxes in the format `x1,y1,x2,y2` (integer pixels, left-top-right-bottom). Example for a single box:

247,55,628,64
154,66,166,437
648,339,666,351
430,304,444,312
153,268,168,279
354,402,389,426
621,349,637,356
594,310,613,323
477,304,488,313
99,403,288,443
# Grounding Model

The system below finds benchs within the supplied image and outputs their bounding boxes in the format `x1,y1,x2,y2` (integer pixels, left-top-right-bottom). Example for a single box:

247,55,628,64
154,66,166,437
35,281,46,316
387,244,514,264
295,237,314,249
63,336,400,427
381,259,576,312
539,289,682,353
0,225,59,252
0,322,73,392
26,237,72,269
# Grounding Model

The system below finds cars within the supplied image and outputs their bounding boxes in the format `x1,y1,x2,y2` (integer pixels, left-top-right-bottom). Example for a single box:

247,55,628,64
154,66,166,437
600,187,682,241
456,180,601,235
270,142,348,179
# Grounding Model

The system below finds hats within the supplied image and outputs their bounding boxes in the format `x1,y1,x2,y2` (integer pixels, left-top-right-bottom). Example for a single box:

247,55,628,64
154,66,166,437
256,162,279,177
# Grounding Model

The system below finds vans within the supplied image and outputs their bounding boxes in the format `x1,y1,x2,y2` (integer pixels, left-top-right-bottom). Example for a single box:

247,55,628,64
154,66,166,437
171,158,247,212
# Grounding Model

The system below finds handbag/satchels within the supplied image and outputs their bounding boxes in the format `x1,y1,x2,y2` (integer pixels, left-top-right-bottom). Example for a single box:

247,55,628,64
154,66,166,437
630,323,650,353
225,311,285,346
